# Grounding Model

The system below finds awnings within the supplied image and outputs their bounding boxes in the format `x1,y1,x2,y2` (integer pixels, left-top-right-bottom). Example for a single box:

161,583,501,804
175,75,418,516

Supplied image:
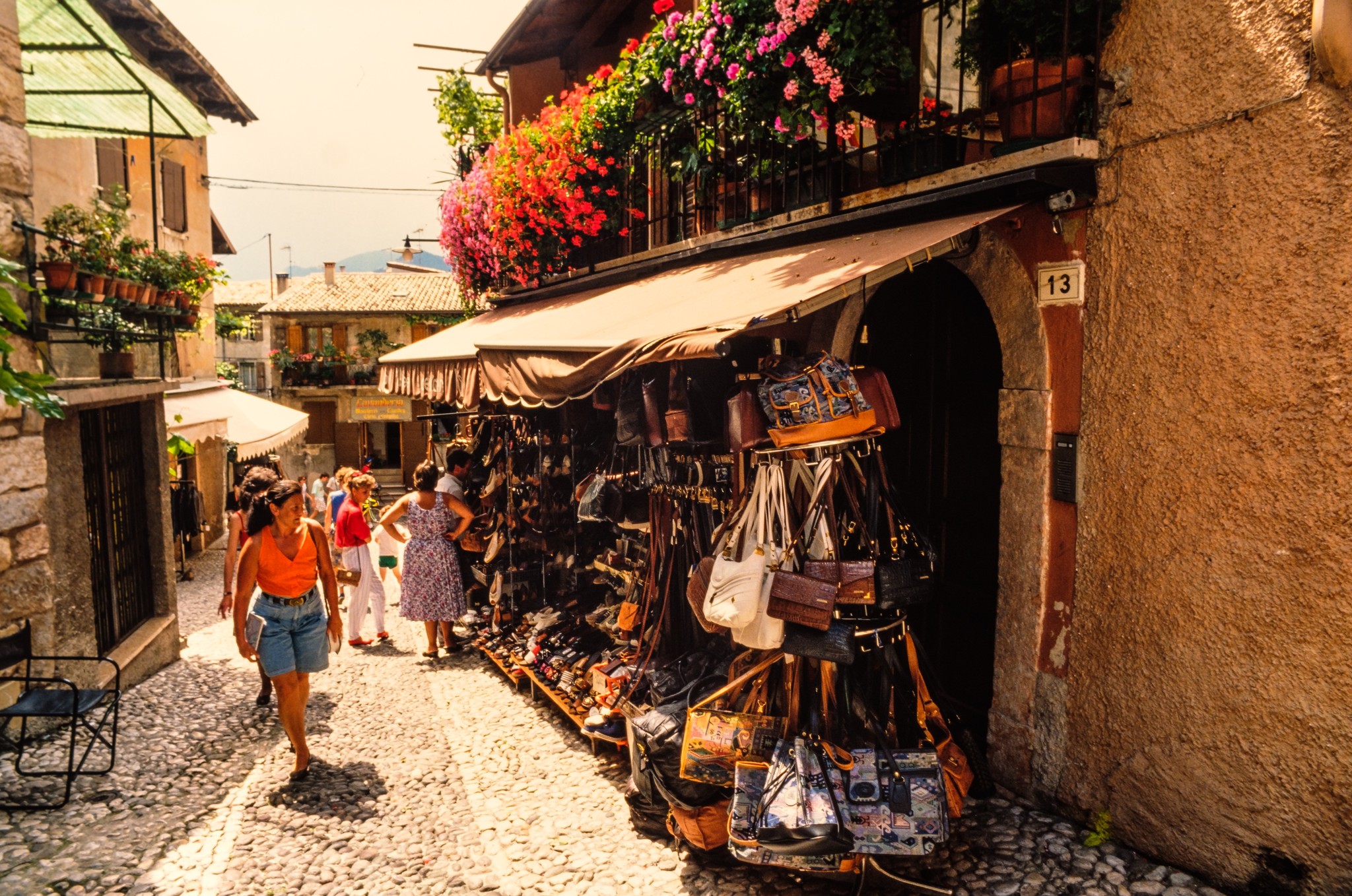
378,205,1026,409
16,0,215,141
163,387,310,463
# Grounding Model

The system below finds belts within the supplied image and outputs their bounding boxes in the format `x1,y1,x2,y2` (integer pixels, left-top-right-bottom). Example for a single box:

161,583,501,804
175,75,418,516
607,454,733,713
262,584,317,607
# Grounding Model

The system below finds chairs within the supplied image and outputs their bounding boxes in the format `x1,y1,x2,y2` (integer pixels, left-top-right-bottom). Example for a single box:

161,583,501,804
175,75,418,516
0,619,121,811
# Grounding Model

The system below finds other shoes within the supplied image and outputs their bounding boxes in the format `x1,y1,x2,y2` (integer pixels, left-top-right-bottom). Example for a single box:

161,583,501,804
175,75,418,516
444,644,463,654
479,433,595,570
348,636,375,646
289,750,311,780
377,631,388,638
338,593,345,605
290,741,311,763
454,570,647,743
422,650,439,658
257,689,272,706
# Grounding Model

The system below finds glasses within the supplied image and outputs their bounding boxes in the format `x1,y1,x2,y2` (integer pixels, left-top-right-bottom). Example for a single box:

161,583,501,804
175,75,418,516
303,479,306,481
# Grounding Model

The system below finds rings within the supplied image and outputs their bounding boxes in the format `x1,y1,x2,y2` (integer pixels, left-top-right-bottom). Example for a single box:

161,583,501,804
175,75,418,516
220,606,224,609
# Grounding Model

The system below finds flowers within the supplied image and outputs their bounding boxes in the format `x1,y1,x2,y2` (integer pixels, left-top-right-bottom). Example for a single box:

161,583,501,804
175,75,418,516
268,345,318,374
43,203,231,305
436,0,962,318
339,347,359,365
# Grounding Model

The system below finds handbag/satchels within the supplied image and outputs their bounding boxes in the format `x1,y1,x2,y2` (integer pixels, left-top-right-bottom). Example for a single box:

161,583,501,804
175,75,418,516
460,528,486,552
574,447,655,524
614,360,696,447
684,448,937,665
336,535,362,588
325,627,342,655
624,639,975,880
727,350,901,453
616,601,642,631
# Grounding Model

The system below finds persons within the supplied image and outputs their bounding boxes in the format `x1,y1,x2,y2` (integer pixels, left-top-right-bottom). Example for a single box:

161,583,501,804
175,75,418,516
297,456,407,605
435,449,489,647
334,469,389,645
234,479,343,780
379,462,474,657
218,468,279,705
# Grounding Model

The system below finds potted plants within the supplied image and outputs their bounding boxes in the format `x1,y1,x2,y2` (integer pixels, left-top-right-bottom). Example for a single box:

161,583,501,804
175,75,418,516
77,300,161,378
361,349,370,364
322,340,341,362
953,0,1120,140
747,156,794,217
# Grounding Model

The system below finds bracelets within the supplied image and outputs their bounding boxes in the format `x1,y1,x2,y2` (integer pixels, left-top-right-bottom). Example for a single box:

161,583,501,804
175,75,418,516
223,592,232,595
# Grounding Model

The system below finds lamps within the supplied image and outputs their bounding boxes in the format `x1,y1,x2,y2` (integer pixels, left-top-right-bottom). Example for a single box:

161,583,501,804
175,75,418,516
392,234,439,260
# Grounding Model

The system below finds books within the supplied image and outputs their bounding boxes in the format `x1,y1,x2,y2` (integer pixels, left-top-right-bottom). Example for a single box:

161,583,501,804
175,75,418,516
233,609,264,655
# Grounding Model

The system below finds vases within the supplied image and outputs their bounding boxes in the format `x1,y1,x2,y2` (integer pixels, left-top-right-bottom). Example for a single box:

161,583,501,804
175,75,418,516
879,134,968,186
104,278,118,298
717,180,746,225
127,281,200,328
40,260,79,289
78,270,105,295
116,278,129,299
